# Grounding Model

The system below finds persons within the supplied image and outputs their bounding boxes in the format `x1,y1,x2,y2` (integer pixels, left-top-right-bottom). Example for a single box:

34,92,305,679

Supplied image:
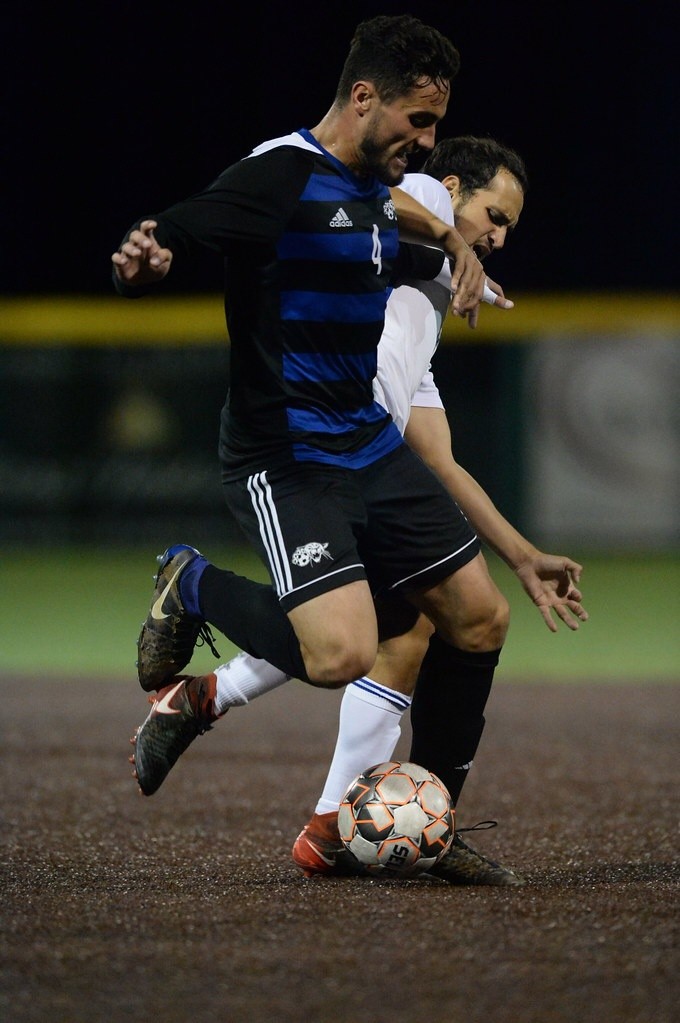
111,13,590,889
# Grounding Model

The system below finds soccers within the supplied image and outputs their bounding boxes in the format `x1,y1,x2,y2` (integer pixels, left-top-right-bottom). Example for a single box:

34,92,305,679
338,760,458,879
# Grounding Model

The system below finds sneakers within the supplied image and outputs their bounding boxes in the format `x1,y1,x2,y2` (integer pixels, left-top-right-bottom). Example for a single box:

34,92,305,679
130,674,229,796
136,543,220,692
292,811,346,877
426,820,523,885
